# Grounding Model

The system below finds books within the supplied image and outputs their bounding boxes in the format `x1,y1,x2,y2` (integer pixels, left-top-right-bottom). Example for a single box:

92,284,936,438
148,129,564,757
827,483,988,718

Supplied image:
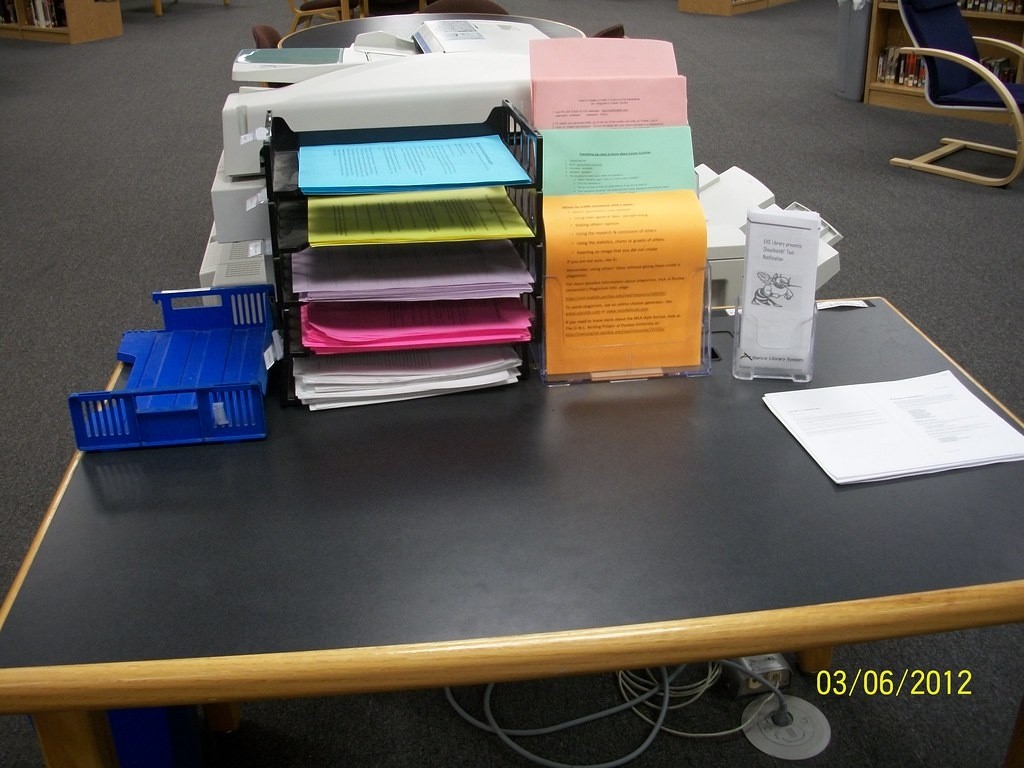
0,0,67,28
981,57,1017,84
877,46,926,88
957,0,1024,14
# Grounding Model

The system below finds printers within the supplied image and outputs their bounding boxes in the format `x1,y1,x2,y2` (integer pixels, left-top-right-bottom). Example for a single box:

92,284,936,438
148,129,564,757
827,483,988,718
199,18,550,287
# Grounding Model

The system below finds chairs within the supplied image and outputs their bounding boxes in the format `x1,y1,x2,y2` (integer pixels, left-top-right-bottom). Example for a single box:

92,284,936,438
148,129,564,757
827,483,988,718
288,0,368,35
888,0,1024,187
252,26,282,87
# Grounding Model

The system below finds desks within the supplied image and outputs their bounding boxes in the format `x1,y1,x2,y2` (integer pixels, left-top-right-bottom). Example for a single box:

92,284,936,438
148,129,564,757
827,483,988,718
0,296,1024,768
278,12,586,48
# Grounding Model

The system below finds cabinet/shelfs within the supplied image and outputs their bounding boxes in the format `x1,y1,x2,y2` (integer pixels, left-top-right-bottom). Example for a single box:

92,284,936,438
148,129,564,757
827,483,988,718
863,0,1024,127
0,0,124,45
261,98,546,408
